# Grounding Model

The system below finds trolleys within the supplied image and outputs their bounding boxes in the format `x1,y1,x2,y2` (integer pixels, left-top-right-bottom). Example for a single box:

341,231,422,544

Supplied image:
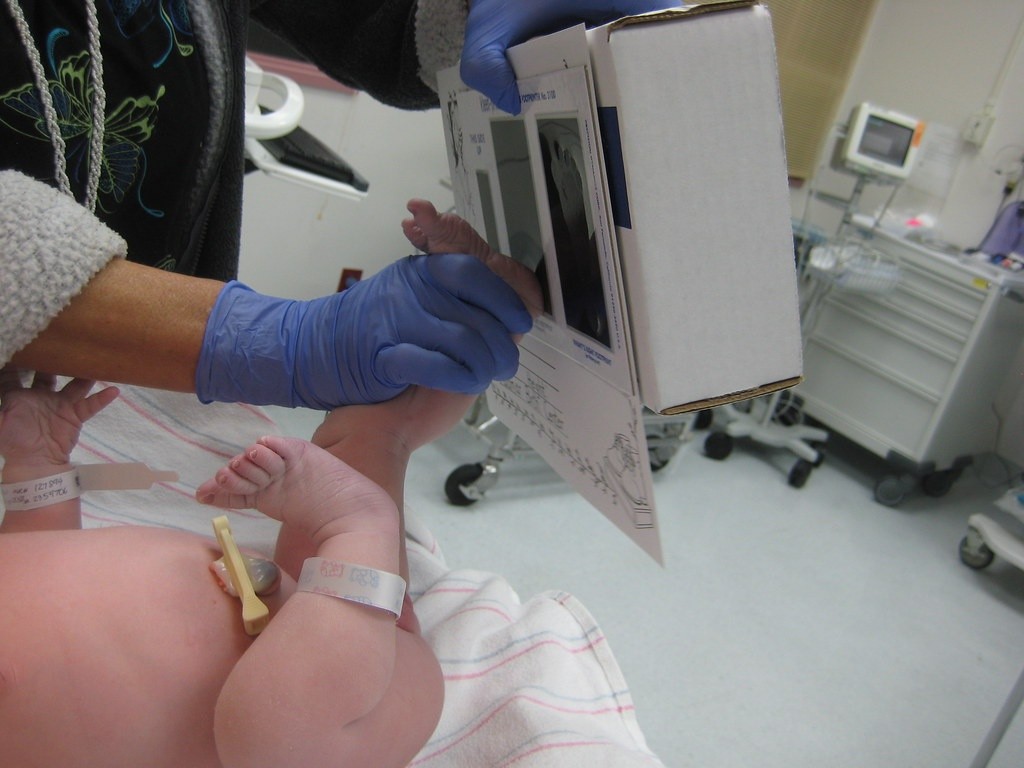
443,392,697,506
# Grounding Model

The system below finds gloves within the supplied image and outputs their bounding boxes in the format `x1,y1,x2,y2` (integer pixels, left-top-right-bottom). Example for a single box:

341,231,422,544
460,0,686,116
195,253,534,410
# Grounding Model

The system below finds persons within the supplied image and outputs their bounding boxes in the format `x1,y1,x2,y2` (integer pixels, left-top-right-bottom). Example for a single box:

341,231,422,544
0,0,680,410
0,200,542,768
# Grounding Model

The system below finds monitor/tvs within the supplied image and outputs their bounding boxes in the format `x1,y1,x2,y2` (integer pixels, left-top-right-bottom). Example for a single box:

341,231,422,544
840,103,924,180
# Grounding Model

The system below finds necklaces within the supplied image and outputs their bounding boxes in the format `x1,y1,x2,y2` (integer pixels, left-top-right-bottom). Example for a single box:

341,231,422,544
8,0,106,215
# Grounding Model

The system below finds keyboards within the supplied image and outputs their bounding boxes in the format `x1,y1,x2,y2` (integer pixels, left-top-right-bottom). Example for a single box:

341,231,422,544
256,102,355,184
